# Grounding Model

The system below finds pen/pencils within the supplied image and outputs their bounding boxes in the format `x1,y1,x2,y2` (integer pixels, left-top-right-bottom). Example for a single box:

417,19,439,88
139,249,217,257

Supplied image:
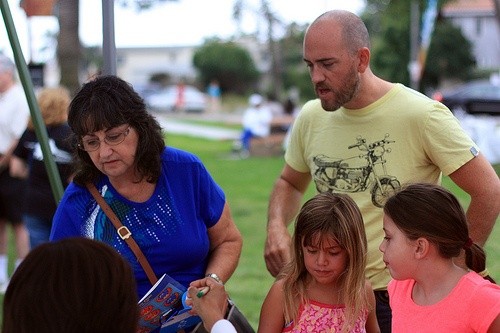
195,287,210,299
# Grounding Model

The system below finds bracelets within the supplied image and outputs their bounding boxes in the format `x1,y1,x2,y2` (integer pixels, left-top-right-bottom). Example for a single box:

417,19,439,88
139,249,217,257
205,273,224,286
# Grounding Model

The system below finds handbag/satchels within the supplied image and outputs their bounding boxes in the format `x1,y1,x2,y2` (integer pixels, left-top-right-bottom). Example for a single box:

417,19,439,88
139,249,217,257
191,291,255,333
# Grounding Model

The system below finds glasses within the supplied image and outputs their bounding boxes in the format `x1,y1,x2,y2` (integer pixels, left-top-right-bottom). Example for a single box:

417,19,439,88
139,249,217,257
78,125,132,152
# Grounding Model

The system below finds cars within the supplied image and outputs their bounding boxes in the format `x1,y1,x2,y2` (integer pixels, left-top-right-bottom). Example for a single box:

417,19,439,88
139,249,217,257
430,78,500,120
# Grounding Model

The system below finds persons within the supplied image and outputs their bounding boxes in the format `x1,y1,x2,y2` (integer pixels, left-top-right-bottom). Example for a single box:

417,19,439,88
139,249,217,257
49,75,242,316
2,237,238,333
380,184,500,333
0,56,32,294
10,86,79,249
264,9,500,333
230,93,270,159
257,192,380,333
